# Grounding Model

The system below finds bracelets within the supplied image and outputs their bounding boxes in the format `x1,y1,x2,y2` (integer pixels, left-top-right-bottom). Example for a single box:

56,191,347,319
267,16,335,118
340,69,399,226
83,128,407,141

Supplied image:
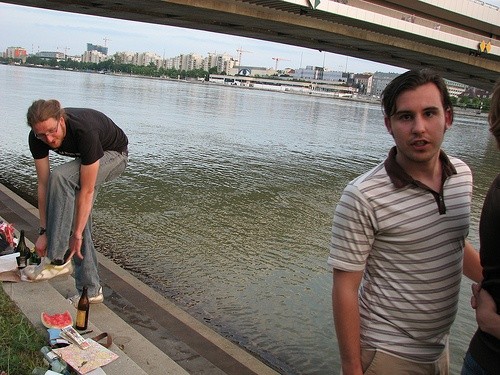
38,226,46,235
68,235,84,241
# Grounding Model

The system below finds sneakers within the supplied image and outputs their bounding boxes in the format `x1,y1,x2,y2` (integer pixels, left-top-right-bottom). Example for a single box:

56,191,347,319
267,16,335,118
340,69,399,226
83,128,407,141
20,257,74,283
66,286,105,309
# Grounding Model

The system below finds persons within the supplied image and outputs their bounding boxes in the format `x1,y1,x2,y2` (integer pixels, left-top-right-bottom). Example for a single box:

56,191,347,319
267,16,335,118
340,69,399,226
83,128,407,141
23,99,128,306
461,80,500,375
327,69,482,375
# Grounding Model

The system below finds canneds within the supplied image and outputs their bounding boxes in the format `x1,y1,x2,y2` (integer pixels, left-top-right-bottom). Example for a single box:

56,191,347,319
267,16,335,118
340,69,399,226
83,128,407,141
29,249,41,265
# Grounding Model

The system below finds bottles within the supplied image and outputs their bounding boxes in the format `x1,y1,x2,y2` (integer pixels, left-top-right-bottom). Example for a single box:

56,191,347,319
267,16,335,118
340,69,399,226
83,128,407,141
32,366,64,375
39,346,73,375
15,230,42,270
75,286,90,331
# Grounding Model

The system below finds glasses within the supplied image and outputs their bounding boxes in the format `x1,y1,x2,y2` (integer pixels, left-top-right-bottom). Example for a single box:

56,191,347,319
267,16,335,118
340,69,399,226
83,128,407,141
34,118,58,140
